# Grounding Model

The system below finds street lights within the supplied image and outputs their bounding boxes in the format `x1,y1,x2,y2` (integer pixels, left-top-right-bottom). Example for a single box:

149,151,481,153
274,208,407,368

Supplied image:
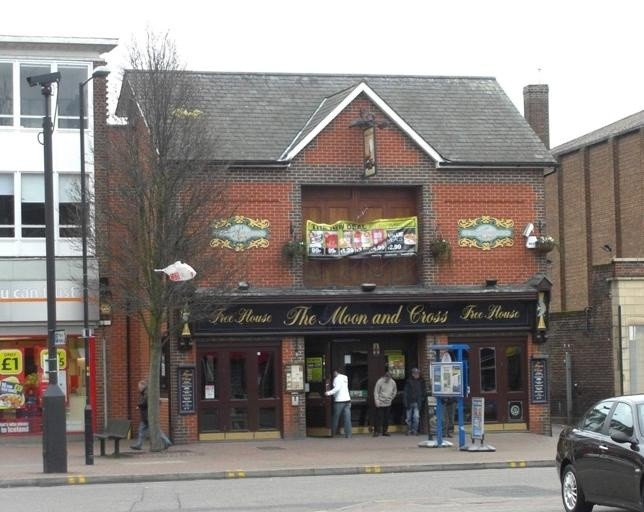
77,66,110,465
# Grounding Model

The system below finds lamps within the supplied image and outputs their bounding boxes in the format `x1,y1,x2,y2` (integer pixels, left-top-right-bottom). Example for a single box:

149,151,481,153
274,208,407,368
532,314,548,343
238,282,249,289
486,279,498,286
361,284,377,291
600,244,616,261
178,319,194,352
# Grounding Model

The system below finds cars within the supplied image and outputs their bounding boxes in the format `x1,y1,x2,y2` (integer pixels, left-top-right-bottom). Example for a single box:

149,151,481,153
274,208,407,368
555,393,644,512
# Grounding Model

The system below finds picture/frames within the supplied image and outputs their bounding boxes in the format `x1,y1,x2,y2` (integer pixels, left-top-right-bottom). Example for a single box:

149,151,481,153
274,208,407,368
284,363,305,393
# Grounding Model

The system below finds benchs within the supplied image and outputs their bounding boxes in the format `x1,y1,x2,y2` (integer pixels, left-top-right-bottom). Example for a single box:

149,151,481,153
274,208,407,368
94,418,133,457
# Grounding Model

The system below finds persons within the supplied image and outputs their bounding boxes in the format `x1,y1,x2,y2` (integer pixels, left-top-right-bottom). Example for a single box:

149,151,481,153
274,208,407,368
130,380,172,451
442,399,456,438
402,368,426,437
372,372,398,437
325,367,353,439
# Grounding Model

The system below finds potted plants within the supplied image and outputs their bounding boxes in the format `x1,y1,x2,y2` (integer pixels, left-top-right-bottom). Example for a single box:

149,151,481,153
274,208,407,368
429,225,453,259
281,231,304,257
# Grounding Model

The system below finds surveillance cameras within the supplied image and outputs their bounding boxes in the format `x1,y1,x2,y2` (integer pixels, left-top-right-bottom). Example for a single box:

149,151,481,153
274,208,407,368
26,71,61,87
521,224,534,238
